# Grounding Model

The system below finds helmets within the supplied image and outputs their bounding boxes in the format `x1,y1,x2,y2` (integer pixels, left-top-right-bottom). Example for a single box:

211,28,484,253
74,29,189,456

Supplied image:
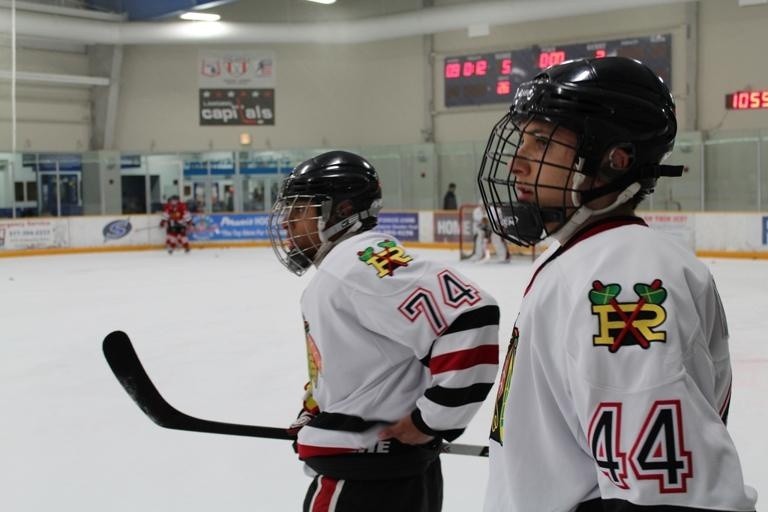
477,53,685,249
265,151,384,278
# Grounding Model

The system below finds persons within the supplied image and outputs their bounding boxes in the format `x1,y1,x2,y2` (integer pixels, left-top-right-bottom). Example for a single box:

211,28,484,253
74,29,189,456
267,151,500,512
470,198,511,263
443,182,457,208
159,195,192,255
477,55,757,511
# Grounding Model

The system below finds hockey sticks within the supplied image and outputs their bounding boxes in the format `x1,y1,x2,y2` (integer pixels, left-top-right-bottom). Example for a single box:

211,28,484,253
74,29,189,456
102,331,489,457
